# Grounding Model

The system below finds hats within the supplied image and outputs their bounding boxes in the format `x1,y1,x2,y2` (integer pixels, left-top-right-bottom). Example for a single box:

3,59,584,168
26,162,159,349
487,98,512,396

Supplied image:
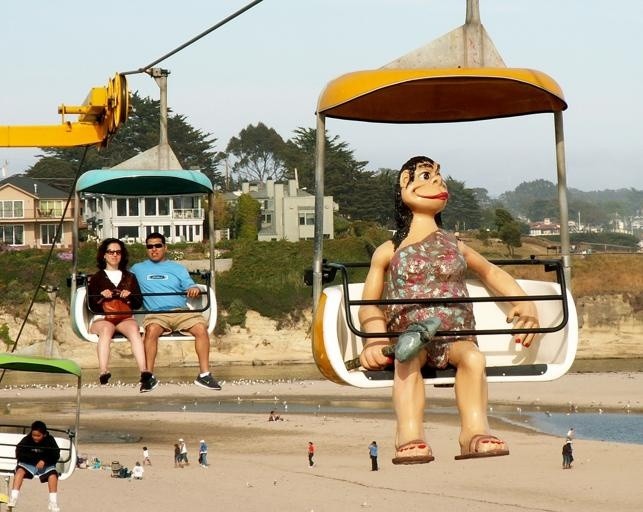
199,440,204,444
178,439,184,442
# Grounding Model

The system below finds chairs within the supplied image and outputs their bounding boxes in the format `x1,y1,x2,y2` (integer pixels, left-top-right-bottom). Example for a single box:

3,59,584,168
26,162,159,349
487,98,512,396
111,461,120,476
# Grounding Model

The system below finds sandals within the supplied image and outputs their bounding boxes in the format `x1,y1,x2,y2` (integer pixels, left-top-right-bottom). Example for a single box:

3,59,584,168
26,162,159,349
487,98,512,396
139,370,151,383
98,371,111,385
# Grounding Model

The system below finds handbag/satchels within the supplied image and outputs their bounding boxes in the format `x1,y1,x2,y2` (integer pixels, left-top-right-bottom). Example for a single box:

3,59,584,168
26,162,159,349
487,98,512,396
96,289,132,324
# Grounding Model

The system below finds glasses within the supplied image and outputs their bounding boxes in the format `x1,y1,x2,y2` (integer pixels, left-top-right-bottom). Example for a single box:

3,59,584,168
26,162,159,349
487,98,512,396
146,244,162,249
105,250,120,255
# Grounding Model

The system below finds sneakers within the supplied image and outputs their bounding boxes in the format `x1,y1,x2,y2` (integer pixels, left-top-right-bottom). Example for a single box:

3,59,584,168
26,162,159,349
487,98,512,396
47,502,60,512
6,496,17,508
139,376,159,392
193,373,220,390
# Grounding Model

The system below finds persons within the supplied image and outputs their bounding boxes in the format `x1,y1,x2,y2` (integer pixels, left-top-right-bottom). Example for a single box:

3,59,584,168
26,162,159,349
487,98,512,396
368,441,378,470
6,421,62,512
269,411,284,421
128,438,208,479
308,442,315,468
129,232,222,393
562,428,574,469
88,238,152,385
360,156,540,464
